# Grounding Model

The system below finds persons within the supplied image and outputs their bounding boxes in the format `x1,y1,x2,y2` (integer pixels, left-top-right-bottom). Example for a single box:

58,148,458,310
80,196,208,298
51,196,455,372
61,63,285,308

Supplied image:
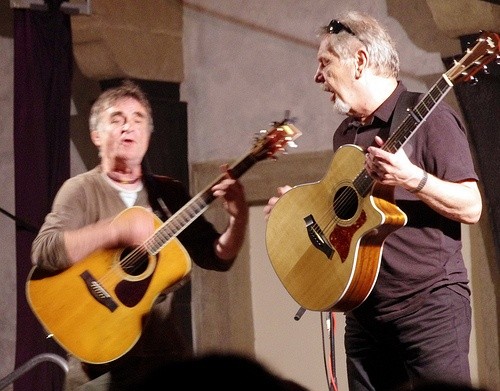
263,11,483,390
30,79,250,391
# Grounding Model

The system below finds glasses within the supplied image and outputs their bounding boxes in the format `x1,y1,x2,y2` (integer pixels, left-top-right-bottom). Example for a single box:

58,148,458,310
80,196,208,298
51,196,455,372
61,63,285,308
327,19,369,51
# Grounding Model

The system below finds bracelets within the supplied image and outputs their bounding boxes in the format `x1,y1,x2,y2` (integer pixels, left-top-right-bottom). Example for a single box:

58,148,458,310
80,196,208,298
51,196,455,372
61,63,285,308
407,169,428,193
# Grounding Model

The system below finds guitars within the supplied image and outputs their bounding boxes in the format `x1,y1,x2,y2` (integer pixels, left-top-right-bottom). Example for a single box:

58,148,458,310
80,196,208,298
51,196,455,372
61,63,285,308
266,30,500,313
25,110,303,365
